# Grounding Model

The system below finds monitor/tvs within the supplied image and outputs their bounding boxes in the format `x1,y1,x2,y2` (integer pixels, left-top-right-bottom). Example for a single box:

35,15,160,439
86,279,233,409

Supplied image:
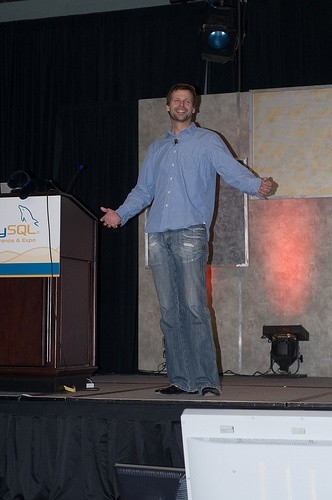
113,463,189,500
0,182,23,194
180,408,332,500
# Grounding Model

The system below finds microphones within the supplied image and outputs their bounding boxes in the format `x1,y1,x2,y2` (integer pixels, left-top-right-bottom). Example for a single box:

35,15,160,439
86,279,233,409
65,164,86,193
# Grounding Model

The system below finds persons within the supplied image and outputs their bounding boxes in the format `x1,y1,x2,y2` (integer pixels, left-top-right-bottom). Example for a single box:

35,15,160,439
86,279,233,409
100,83,272,397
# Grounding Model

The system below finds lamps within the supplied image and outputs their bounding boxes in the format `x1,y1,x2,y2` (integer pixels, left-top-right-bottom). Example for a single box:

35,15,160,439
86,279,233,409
261,325,309,377
199,0,247,64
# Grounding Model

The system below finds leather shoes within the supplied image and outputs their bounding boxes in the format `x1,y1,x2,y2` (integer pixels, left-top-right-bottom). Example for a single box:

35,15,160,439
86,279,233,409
202,387,220,396
155,385,199,394
